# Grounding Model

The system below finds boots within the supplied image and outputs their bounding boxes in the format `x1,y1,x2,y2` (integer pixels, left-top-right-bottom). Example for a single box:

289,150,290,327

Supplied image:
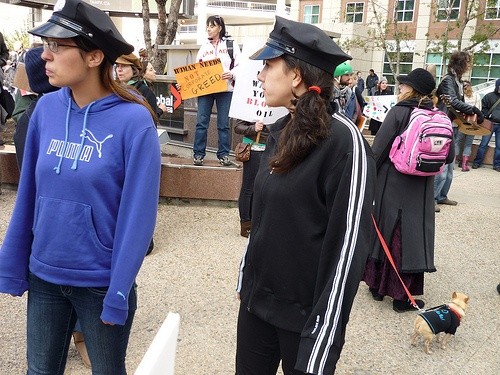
461,155,471,171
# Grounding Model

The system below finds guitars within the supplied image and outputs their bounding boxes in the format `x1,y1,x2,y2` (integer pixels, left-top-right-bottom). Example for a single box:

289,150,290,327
440,94,493,136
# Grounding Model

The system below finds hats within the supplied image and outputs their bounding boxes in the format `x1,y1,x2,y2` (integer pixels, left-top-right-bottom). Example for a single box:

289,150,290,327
24,48,61,93
249,15,353,75
116,52,138,65
27,0,134,64
396,68,436,95
378,76,387,84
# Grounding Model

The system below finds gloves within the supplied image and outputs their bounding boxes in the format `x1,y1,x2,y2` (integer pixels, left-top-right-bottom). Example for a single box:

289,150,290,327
472,106,484,124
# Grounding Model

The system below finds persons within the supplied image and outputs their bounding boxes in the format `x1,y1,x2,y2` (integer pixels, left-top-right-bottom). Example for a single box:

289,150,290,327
234,119,270,238
0,33,9,145
235,17,378,375
360,68,437,312
0,0,162,375
175,15,242,166
15,45,93,367
433,51,485,212
115,44,167,257
453,79,500,172
331,62,394,135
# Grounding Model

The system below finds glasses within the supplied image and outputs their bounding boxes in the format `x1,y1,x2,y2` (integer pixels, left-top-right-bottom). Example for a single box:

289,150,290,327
215,15,222,25
41,37,90,53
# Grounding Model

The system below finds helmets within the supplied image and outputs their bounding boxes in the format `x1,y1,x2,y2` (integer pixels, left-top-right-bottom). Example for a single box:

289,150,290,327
333,62,353,78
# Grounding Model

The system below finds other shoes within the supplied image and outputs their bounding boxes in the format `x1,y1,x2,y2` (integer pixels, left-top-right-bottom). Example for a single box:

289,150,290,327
73,331,92,369
393,299,425,313
369,287,384,301
435,200,440,212
472,162,480,169
437,197,458,206
493,167,500,172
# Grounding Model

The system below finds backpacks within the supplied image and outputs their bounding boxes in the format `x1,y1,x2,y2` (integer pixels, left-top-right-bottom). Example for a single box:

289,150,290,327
386,106,454,177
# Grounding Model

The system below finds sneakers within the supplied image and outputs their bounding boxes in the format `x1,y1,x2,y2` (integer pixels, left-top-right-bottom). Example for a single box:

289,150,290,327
193,155,204,165
219,156,231,166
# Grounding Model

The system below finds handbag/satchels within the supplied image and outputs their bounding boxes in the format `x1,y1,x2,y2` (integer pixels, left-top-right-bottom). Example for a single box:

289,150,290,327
235,143,252,162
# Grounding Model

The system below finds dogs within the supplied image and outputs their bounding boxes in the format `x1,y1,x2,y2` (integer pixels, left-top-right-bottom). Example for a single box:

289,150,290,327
410,291,470,355
